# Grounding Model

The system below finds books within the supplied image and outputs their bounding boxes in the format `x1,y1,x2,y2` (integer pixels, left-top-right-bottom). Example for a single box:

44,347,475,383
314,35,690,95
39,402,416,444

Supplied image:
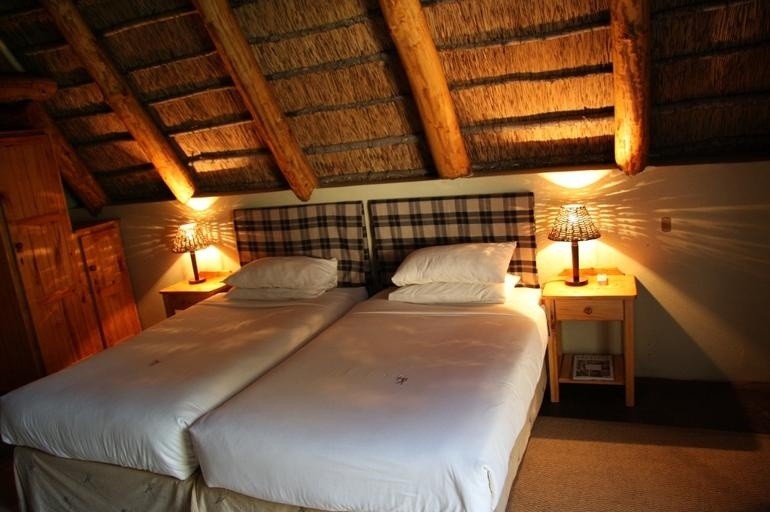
573,354,614,381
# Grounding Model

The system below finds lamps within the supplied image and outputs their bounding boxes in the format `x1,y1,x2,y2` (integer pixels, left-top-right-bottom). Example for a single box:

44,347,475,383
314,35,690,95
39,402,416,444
548,205,599,287
170,220,212,285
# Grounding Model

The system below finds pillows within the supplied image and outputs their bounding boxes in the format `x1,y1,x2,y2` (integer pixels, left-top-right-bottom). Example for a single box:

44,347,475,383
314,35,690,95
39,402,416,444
221,256,337,290
226,284,327,299
393,242,516,288
388,274,522,306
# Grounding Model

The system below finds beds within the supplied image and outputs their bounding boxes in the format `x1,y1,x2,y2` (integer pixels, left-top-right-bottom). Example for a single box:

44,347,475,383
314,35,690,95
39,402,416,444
0,200,369,512
190,190,549,512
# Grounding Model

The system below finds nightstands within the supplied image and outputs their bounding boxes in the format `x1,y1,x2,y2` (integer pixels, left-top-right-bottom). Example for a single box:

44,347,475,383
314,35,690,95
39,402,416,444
542,274,639,409
160,276,234,319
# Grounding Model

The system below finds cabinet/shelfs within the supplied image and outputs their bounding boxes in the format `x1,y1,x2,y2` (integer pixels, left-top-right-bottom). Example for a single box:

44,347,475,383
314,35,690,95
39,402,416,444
0,132,105,380
73,216,143,352
